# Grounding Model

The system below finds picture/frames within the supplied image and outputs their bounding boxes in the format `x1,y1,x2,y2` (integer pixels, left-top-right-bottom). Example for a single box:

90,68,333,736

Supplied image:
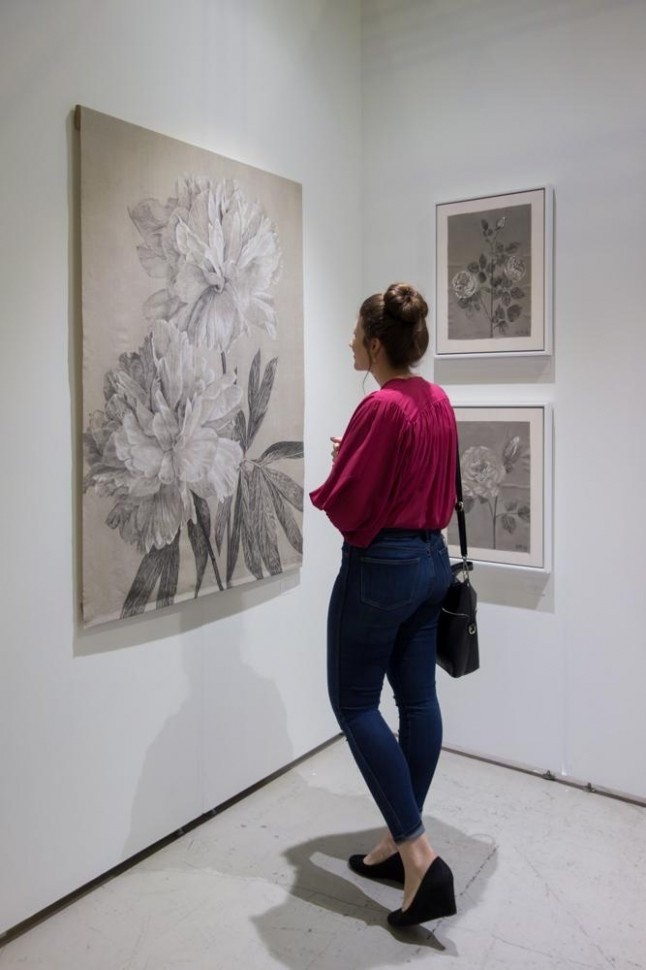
439,400,557,573
428,182,556,358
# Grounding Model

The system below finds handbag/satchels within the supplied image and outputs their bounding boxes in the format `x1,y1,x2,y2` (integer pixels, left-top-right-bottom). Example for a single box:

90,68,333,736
436,561,480,678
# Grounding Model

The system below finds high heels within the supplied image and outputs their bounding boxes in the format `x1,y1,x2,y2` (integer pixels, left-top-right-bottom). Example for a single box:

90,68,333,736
348,851,405,885
387,855,458,928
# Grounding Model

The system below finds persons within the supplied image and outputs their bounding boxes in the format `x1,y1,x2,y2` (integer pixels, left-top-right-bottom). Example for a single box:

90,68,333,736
309,283,480,927
